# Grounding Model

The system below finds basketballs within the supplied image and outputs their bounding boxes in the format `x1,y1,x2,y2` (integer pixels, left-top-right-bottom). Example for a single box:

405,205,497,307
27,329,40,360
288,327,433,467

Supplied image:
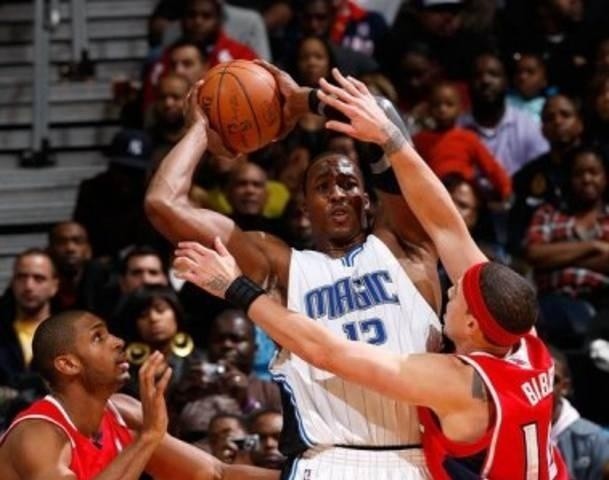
197,58,283,157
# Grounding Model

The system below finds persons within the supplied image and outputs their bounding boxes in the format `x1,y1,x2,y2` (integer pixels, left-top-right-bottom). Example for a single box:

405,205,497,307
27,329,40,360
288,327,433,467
0,1,608,479
